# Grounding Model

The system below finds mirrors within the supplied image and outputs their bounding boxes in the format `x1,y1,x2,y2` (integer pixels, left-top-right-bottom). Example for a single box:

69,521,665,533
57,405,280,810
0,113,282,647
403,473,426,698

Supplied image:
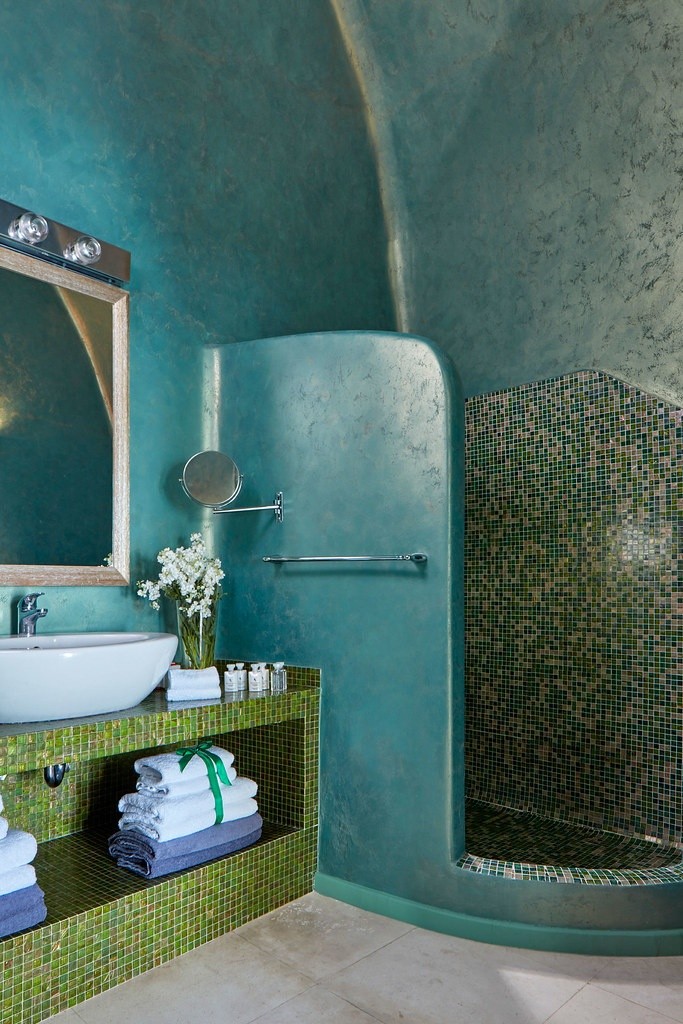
1,242,132,588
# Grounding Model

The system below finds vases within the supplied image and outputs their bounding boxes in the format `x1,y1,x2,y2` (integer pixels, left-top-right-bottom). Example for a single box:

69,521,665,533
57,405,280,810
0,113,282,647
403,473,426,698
135,533,225,669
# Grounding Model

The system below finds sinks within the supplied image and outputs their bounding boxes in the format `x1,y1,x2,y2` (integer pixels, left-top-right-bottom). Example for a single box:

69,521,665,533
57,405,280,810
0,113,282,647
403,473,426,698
0,628,180,724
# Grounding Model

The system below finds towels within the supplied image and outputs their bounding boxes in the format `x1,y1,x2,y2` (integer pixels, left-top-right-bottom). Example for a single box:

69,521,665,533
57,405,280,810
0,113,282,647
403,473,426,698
164,687,223,702
167,665,221,689
0,785,48,939
107,741,263,880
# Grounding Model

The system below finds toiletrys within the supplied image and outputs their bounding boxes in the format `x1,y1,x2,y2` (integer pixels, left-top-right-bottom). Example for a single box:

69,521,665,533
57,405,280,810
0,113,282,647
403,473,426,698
274,661,287,689
234,662,248,691
248,664,263,692
270,663,285,692
255,662,271,690
224,664,239,693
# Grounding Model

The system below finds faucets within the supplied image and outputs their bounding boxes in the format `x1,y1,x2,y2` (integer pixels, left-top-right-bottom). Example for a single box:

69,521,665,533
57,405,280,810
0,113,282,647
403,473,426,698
15,590,50,636
